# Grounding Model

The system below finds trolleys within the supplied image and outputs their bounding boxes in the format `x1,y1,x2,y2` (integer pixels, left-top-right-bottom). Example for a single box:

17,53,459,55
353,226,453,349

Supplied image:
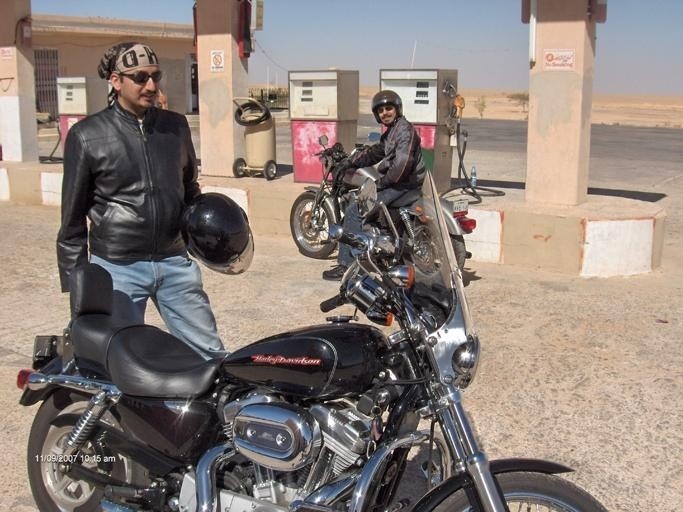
233,95,277,179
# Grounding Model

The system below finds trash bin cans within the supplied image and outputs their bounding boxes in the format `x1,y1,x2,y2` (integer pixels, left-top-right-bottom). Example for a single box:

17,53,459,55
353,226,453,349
268,94,276,103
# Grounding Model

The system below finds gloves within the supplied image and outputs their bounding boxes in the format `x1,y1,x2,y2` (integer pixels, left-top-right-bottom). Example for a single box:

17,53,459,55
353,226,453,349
334,159,353,171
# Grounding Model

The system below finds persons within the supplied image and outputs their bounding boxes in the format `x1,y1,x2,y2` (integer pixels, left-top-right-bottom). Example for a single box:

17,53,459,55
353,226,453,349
323,90,426,281
56,43,231,361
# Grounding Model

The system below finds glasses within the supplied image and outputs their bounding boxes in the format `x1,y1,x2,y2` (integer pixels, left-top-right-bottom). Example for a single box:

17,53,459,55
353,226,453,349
121,69,164,85
378,106,393,113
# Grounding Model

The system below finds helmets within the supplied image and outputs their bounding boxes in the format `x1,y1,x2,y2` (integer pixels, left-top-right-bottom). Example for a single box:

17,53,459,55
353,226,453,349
178,192,255,276
372,89,404,125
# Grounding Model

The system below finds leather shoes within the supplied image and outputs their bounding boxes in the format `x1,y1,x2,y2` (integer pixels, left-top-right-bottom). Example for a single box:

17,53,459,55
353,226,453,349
323,265,348,281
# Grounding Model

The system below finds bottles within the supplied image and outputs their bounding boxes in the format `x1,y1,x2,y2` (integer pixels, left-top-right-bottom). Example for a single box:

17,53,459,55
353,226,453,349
470,167,477,188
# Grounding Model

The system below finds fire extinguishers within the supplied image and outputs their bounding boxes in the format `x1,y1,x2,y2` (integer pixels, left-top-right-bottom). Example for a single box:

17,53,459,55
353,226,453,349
14,14,32,48
192,2,196,46
